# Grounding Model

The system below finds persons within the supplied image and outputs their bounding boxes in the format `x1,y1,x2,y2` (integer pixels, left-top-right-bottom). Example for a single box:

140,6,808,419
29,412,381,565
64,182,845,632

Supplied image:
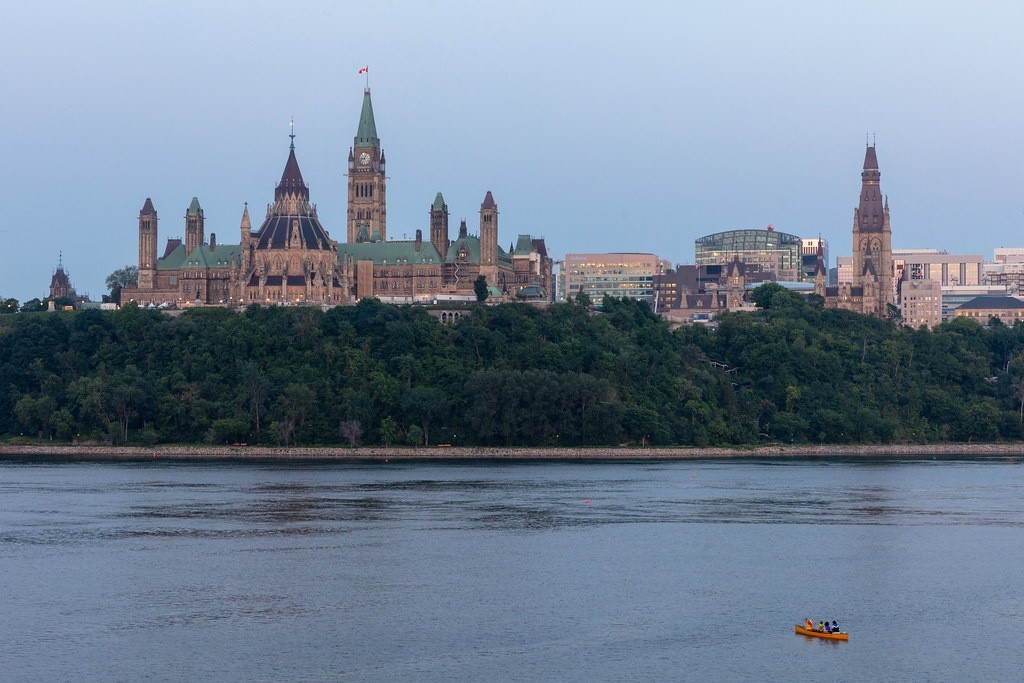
804,618,840,634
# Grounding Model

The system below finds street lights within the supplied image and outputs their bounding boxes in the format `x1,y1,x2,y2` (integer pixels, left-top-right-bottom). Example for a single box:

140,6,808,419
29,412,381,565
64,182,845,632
230,297,232,307
179,298,182,308
328,294,330,305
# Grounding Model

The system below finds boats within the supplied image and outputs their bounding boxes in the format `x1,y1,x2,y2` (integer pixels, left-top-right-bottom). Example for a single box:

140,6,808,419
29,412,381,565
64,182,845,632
794,624,849,641
